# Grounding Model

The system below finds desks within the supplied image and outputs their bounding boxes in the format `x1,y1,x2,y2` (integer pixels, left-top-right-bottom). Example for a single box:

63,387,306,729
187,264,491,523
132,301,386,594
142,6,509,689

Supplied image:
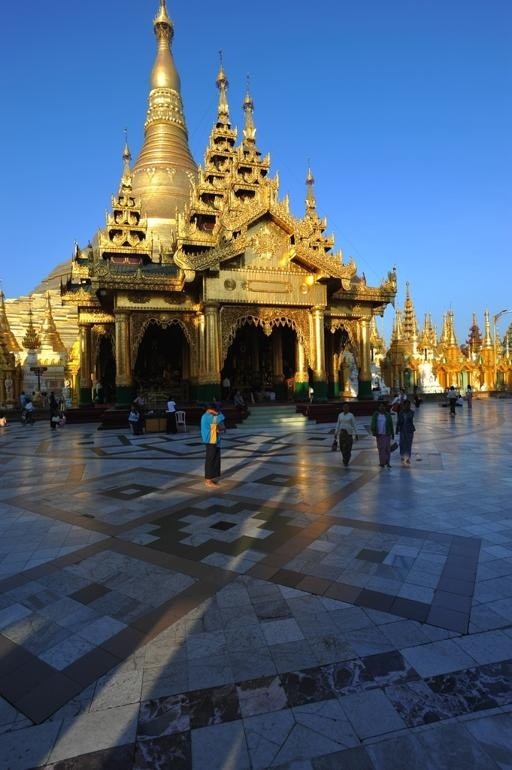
142,417,168,434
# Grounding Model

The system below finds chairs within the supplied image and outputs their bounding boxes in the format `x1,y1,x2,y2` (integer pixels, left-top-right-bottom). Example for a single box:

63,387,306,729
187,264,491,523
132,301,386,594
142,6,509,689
175,411,187,432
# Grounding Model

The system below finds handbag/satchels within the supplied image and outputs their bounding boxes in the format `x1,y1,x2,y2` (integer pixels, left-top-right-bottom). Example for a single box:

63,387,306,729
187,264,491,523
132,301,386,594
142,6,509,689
390,442,398,452
332,440,337,451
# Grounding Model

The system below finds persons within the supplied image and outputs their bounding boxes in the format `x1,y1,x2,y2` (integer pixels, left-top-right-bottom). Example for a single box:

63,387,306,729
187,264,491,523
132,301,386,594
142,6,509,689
397,400,416,465
166,396,178,435
446,386,458,416
20,391,66,431
334,402,359,467
134,393,147,405
456,395,462,406
466,385,472,408
200,402,226,488
221,375,251,420
397,389,408,416
5,375,13,392
370,402,395,468
